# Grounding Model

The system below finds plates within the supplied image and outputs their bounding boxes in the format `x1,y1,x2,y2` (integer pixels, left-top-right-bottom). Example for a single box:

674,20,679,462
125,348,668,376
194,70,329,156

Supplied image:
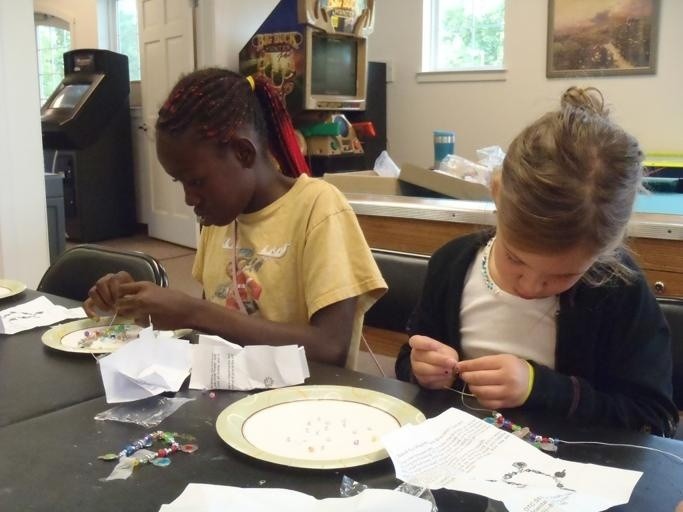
0,276,28,302
214,383,428,472
39,314,194,355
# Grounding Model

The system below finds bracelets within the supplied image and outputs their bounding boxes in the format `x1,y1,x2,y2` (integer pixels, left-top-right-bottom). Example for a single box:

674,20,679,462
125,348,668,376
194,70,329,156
96,427,200,483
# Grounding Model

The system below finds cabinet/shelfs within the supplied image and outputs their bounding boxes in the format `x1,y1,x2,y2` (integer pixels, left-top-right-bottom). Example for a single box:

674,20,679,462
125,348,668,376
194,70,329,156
341,159,683,418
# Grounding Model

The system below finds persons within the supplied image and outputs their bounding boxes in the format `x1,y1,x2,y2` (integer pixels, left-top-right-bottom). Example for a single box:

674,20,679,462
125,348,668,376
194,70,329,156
395,86,678,438
84,67,390,368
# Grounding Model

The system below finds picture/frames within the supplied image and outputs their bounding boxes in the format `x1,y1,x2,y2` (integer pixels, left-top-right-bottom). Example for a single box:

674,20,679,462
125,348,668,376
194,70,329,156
545,0,662,79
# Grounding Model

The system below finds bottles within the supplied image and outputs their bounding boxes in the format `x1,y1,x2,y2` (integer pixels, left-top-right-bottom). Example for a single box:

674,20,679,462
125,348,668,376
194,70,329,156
433,129,456,172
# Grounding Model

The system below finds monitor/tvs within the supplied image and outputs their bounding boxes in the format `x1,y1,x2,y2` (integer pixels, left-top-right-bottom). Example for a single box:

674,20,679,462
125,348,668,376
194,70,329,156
305,26,368,111
48,84,92,109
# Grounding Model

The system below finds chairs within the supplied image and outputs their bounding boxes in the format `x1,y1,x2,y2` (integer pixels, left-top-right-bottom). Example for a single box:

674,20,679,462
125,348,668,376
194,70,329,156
365,248,432,331
36,243,167,303
655,294,683,442
